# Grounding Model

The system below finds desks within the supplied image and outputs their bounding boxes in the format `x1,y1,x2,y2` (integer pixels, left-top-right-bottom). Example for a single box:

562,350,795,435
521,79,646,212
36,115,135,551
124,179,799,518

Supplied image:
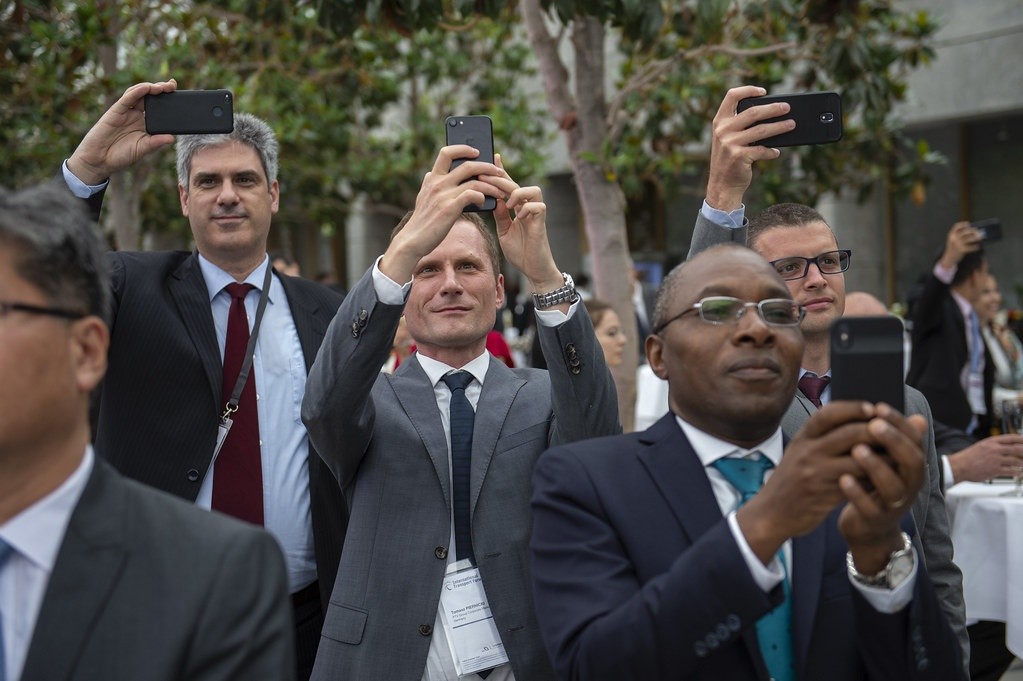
942,475,1023,662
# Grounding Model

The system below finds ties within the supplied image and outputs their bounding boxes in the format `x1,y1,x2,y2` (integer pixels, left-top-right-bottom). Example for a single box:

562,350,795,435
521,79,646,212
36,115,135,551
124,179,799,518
211,282,264,529
707,454,795,681
969,311,979,373
0,535,13,681
798,372,832,410
439,370,495,680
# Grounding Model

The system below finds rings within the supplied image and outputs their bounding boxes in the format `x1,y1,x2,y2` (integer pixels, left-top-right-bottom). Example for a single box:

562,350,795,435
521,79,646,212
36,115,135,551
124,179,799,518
889,489,912,512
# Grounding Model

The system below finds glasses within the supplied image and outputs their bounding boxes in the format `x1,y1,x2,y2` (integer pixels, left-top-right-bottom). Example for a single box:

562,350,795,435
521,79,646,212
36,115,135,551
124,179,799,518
769,249,851,281
653,296,807,335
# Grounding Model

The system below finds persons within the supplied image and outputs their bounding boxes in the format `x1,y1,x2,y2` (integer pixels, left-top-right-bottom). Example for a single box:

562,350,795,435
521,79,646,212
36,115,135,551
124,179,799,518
56,77,347,681
0,178,293,681
526,87,1023,681
300,146,625,680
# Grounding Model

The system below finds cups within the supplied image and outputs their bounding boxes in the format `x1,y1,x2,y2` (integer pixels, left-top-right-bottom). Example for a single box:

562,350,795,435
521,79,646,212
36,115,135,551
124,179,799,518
1002,398,1023,483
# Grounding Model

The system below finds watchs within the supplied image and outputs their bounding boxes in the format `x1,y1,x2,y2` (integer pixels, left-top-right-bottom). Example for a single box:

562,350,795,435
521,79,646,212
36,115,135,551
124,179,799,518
532,271,579,309
847,530,914,590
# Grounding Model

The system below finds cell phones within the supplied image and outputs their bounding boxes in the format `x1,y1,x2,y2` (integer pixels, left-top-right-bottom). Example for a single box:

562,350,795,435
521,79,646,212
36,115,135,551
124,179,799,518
144,89,235,135
737,92,842,148
445,115,497,212
969,218,1003,244
829,316,904,456
